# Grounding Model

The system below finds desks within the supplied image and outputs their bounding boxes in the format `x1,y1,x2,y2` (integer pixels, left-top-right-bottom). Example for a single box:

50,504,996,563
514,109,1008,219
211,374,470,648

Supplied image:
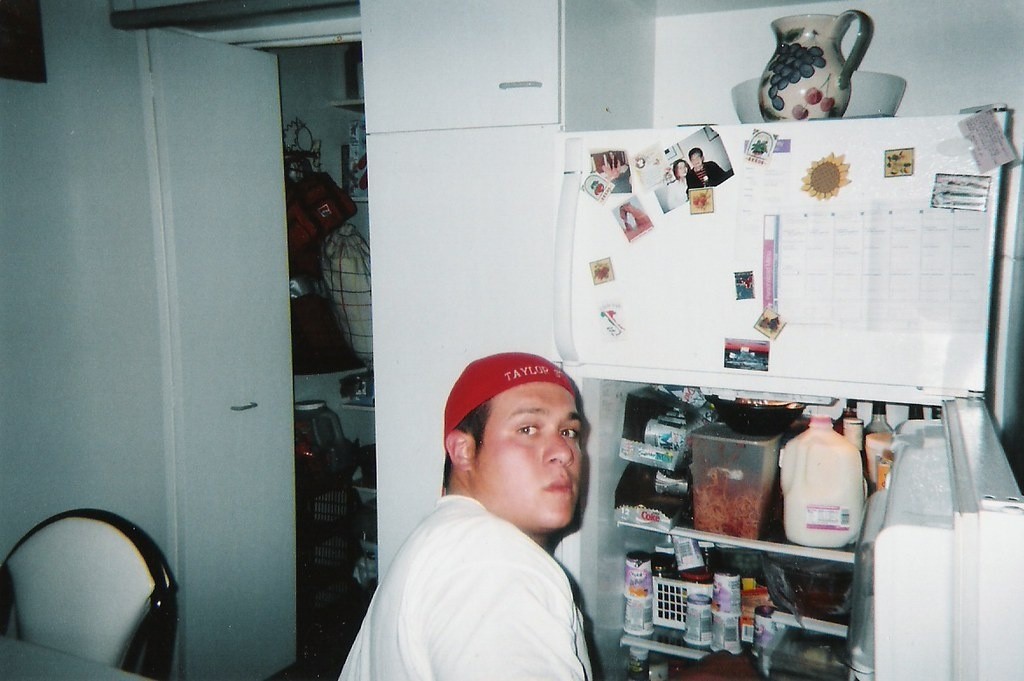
0,635,155,681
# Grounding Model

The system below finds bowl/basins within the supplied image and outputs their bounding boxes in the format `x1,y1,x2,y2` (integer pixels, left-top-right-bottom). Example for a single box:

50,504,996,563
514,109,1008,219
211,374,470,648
704,395,806,437
730,70,906,123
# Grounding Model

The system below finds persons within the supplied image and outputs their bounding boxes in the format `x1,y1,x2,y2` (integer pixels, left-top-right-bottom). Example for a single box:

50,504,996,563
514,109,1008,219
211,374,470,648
338,352,592,681
601,151,621,178
666,148,728,196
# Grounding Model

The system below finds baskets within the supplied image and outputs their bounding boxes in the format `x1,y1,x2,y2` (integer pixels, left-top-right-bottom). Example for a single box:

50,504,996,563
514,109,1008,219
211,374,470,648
651,569,716,632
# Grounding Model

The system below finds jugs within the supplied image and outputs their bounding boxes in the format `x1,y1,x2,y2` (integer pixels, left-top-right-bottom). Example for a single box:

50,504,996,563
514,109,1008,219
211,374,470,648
758,10,874,123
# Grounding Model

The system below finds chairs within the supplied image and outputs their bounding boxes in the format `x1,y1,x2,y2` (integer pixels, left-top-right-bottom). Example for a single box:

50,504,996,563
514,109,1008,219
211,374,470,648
0,508,177,681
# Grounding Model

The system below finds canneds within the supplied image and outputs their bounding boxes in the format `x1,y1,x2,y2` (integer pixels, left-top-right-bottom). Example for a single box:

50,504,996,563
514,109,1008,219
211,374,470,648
679,571,712,611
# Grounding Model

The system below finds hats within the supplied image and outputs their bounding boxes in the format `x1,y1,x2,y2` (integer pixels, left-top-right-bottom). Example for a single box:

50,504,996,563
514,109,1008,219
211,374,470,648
438,351,575,488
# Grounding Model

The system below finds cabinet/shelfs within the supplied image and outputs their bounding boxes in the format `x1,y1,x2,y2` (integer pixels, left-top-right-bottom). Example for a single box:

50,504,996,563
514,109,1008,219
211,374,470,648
340,402,376,496
325,99,370,204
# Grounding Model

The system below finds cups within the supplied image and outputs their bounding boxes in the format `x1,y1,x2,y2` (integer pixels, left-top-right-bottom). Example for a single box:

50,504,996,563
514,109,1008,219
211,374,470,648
622,550,784,658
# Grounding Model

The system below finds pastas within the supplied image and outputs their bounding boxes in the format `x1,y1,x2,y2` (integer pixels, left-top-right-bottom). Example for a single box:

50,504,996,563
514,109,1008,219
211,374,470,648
692,469,776,540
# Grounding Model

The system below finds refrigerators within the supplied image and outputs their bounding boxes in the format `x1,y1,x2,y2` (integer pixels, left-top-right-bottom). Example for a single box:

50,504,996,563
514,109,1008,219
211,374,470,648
548,106,1024,680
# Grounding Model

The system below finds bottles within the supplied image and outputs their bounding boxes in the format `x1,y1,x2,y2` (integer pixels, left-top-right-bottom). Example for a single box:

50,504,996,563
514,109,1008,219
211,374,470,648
623,646,670,681
654,541,738,582
777,399,924,549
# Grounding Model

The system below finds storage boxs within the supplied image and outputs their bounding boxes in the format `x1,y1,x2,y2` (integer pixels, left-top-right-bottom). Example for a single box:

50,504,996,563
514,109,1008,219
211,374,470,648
615,460,692,533
618,386,720,472
690,421,790,541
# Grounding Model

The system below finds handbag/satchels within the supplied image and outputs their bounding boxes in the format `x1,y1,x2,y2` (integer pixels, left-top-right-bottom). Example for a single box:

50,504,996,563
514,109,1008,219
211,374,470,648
284,156,357,254
